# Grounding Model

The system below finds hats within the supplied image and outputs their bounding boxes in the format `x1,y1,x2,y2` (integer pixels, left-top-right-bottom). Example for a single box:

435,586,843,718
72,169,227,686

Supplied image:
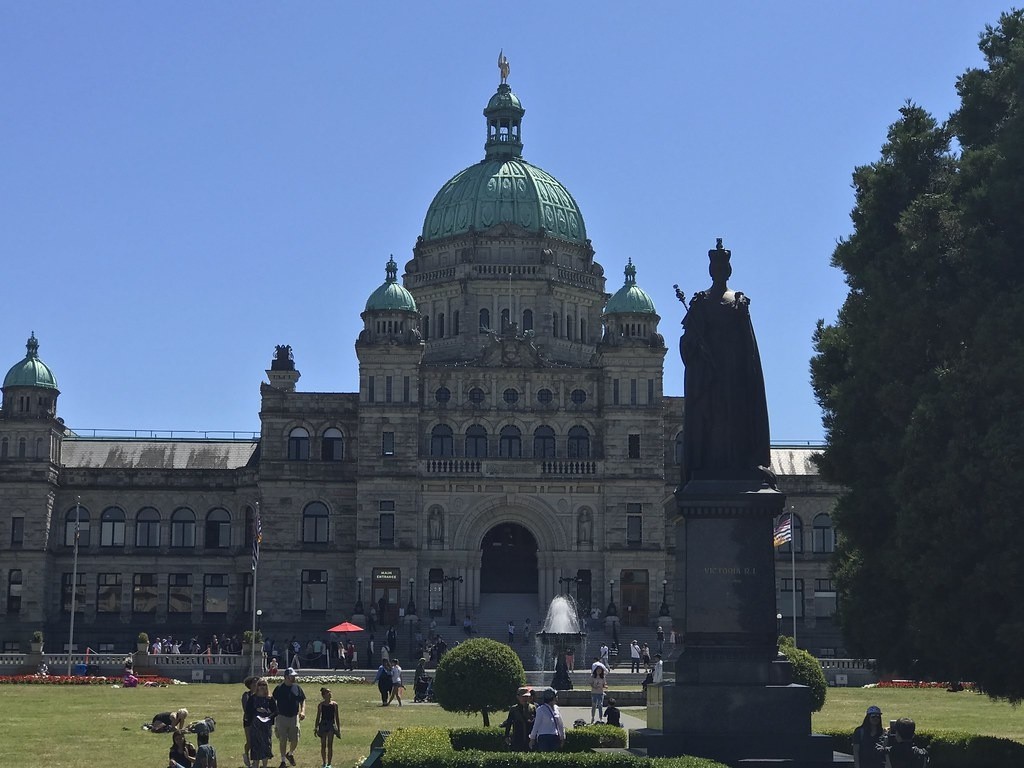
285,667,298,676
244,676,259,689
653,654,661,657
518,688,531,696
543,687,557,703
867,706,882,714
632,640,637,642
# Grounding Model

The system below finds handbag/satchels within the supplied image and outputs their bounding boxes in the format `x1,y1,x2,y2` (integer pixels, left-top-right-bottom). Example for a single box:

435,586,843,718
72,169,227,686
352,652,357,661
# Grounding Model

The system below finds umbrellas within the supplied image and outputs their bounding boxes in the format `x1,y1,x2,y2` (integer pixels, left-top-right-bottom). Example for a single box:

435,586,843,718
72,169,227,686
327,621,365,632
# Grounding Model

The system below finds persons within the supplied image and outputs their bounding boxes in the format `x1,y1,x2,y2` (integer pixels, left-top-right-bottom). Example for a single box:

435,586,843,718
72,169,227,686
151,631,241,664
499,687,566,752
241,675,279,768
268,658,278,677
673,238,772,483
313,687,342,768
36,660,51,675
122,651,141,687
143,708,217,768
505,618,545,646
876,717,930,768
853,706,892,768
272,667,306,768
264,635,357,671
463,616,477,638
604,699,621,727
588,662,609,724
369,595,459,707
550,625,686,691
498,51,510,84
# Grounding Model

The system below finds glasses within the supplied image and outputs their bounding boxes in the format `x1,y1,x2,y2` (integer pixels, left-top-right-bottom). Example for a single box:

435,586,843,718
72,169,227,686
258,683,266,686
870,714,881,718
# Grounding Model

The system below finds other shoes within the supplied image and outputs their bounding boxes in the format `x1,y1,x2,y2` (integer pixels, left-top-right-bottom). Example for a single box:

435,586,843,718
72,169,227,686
279,762,287,768
326,764,331,768
322,764,327,768
243,753,250,766
286,752,296,766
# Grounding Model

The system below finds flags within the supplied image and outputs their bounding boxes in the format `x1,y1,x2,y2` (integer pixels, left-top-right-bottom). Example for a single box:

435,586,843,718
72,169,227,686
72,514,79,555
250,510,262,575
774,519,791,548
